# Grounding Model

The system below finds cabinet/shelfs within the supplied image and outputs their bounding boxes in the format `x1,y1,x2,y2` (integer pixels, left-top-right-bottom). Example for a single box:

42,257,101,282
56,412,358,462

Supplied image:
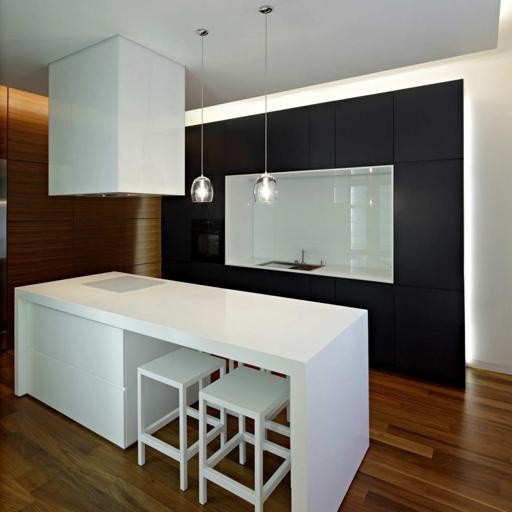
334,81,461,170
185,114,267,178
269,100,334,175
162,180,224,264
392,162,462,291
253,298,255,299
337,282,462,389
163,263,335,305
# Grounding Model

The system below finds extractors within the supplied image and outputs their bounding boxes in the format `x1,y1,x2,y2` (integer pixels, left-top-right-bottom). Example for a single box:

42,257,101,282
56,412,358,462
48,39,185,199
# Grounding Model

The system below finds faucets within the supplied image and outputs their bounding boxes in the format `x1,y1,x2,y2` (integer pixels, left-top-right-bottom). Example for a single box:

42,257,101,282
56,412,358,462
302,248,304,263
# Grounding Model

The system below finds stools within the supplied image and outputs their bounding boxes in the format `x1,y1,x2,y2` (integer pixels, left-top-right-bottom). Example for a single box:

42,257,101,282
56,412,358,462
136,347,292,511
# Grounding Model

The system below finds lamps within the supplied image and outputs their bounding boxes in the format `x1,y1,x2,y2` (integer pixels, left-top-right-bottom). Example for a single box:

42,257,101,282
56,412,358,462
252,7,279,204
190,27,214,203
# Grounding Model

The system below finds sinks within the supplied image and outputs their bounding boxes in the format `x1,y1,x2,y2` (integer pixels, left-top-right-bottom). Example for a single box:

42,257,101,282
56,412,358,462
288,264,325,271
